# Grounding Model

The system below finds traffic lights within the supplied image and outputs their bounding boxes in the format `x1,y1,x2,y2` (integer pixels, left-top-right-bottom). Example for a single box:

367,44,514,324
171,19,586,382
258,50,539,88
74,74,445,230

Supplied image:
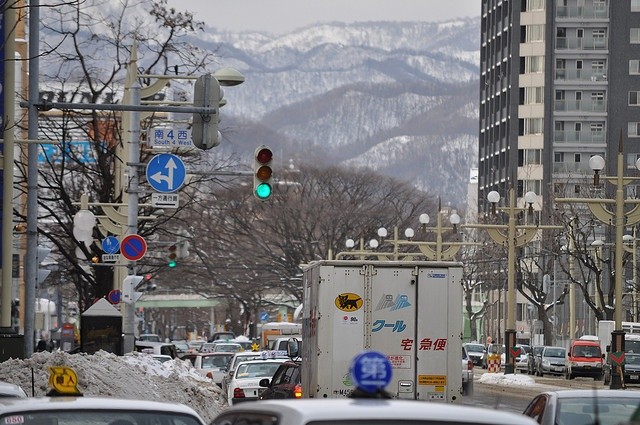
253,143,274,200
167,244,177,267
194,72,224,150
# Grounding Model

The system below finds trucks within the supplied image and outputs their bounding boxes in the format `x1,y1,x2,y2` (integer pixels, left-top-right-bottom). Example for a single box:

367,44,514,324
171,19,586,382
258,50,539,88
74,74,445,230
287,258,464,402
599,319,640,358
262,321,303,347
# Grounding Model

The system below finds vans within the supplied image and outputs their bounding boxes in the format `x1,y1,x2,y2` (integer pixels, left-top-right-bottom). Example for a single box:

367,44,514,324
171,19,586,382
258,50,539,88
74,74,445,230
272,336,302,350
141,333,160,341
135,340,177,358
565,340,605,379
606,333,640,382
528,345,566,375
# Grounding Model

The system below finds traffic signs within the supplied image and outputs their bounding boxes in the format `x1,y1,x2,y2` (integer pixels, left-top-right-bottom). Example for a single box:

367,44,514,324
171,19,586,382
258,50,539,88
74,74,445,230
150,128,192,146
151,192,179,207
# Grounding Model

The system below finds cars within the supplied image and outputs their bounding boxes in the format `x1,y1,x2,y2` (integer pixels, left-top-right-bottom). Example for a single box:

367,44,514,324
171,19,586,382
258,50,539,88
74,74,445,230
499,343,529,372
195,342,215,351
523,388,640,424
213,342,243,351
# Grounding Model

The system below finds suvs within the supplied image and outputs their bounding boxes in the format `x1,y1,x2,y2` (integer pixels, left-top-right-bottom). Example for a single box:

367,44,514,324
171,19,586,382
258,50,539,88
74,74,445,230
207,332,235,341
176,351,235,383
462,342,485,363
258,360,302,398
227,360,286,405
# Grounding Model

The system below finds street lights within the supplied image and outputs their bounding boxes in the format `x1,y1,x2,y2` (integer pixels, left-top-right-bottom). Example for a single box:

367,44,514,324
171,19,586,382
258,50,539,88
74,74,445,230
396,212,481,260
461,189,563,373
554,154,640,388
71,192,152,276
123,38,245,356
369,226,415,260
